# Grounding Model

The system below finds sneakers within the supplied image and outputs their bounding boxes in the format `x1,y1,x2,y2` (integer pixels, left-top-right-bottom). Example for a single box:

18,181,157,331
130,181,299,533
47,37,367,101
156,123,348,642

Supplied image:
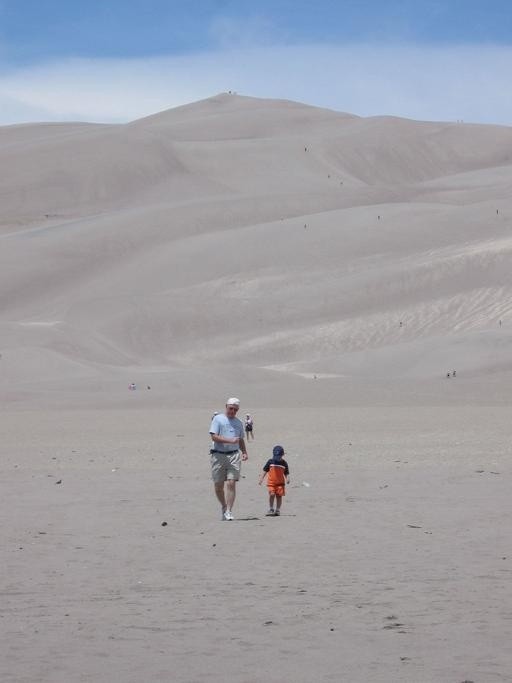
221,504,233,520
266,510,275,515
275,512,280,515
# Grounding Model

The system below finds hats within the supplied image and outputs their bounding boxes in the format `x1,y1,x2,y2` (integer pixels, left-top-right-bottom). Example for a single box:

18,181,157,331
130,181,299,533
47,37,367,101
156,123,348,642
227,398,240,406
274,446,284,455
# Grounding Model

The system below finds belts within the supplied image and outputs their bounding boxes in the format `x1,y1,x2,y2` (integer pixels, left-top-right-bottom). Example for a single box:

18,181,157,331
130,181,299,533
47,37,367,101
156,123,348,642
213,449,238,454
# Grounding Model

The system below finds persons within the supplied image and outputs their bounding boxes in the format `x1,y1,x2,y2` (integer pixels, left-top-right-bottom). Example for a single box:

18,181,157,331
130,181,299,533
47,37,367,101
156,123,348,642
244,414,255,442
131,383,136,391
209,397,249,520
211,411,218,421
145,385,153,391
257,445,291,516
